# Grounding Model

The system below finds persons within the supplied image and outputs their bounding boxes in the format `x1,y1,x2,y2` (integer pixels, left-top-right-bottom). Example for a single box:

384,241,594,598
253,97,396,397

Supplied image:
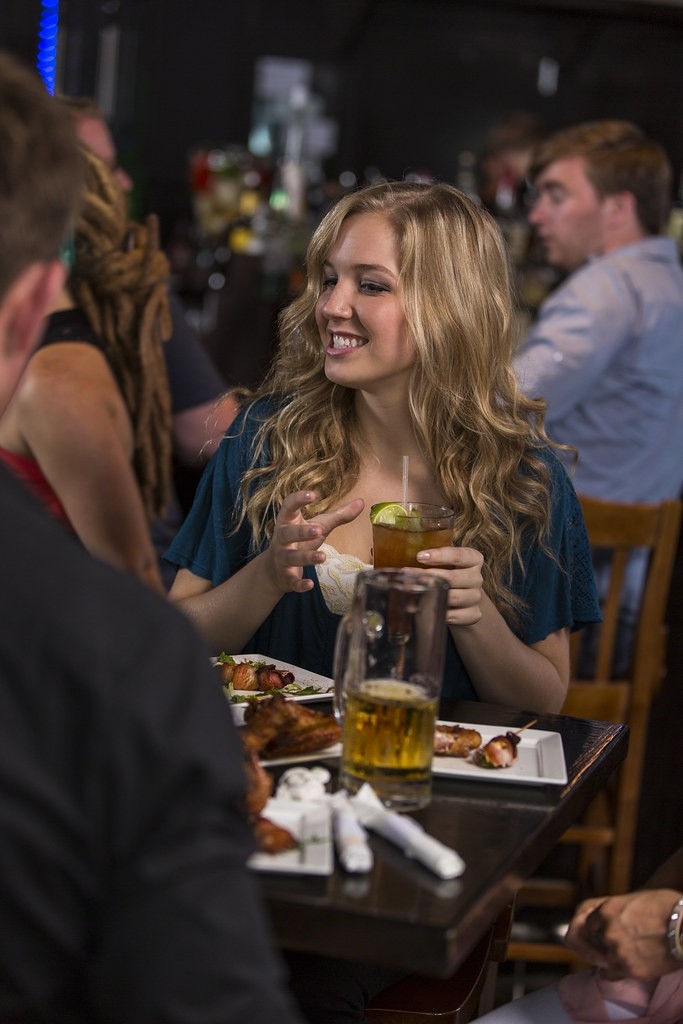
482,116,683,683
0,56,303,1024
469,849,683,1024
164,180,603,713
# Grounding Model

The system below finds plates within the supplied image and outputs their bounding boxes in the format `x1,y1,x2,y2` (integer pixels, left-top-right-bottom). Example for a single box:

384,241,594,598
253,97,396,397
210,654,336,703
431,720,568,786
231,706,343,767
245,797,336,878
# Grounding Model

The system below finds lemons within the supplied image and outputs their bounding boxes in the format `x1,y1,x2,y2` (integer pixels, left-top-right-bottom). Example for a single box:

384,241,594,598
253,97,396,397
370,503,409,524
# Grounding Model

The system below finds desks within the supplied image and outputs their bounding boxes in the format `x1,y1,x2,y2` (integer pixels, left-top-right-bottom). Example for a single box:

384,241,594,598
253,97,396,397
248,690,625,1024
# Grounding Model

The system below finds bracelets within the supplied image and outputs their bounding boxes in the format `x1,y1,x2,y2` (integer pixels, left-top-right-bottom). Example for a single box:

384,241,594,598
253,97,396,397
667,899,683,963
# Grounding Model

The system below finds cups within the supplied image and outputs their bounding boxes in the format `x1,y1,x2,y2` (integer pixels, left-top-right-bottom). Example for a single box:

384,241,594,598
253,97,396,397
332,568,446,813
371,501,455,683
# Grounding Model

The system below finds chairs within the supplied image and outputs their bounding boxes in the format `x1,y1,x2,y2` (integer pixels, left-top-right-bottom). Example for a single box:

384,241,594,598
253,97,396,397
503,496,682,963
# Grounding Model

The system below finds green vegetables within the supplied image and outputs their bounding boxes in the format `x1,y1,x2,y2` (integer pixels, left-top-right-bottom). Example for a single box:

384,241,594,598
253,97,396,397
215,653,323,705
